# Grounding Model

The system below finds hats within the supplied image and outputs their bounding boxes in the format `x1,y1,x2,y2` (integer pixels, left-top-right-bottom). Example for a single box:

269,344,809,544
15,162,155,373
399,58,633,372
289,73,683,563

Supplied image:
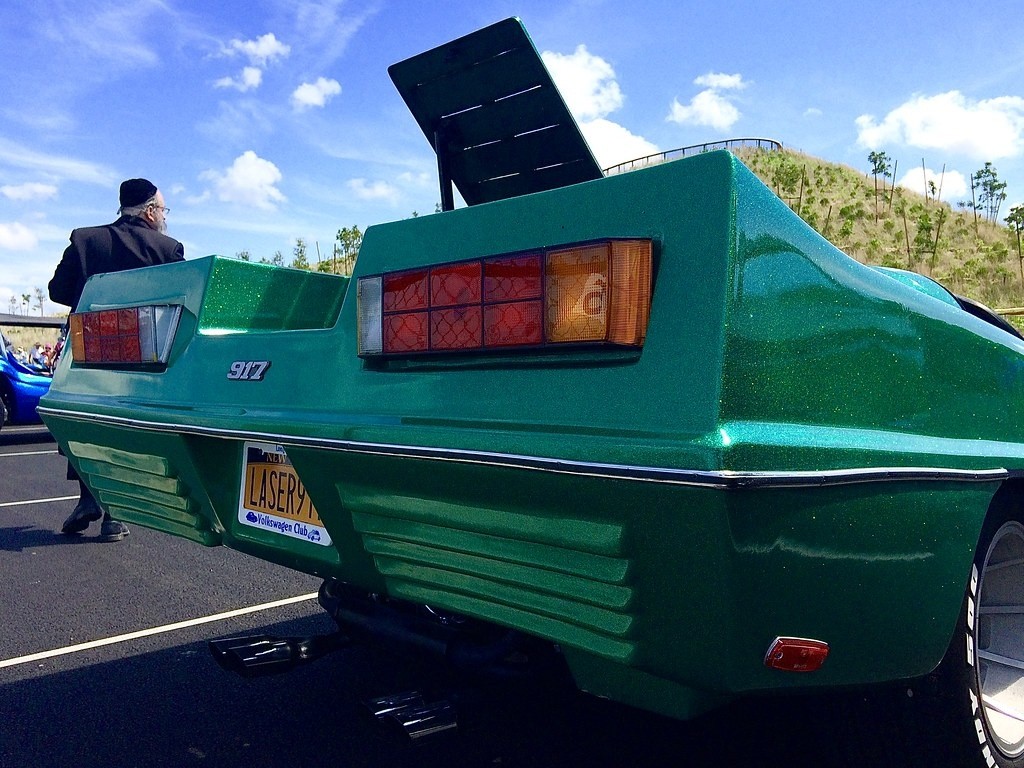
34,342,42,346
119,178,157,209
17,346,23,352
45,343,51,349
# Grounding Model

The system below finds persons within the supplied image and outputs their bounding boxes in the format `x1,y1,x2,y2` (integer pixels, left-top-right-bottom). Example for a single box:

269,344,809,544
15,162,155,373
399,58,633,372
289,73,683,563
17,325,66,369
48,179,186,540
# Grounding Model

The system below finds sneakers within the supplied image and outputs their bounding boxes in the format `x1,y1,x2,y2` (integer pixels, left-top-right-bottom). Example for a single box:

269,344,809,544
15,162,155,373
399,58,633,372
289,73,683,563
100,521,130,541
60,494,102,532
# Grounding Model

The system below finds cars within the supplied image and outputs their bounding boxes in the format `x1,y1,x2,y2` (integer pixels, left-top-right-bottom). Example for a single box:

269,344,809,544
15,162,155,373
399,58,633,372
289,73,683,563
0,329,56,429
27,13,1023,768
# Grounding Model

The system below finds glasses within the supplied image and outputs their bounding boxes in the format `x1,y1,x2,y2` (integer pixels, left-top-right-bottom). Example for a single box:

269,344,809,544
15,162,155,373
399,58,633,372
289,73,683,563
147,204,170,215
